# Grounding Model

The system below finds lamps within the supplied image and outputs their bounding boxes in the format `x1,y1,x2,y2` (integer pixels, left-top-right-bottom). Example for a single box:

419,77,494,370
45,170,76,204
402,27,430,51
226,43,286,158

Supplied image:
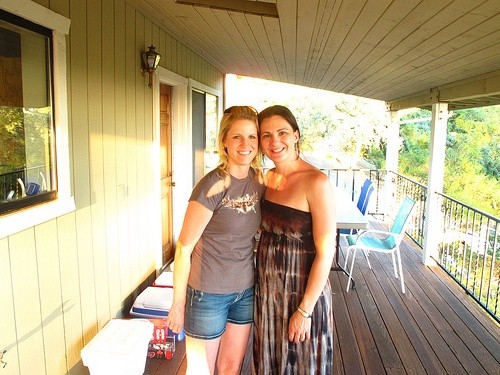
140,44,162,90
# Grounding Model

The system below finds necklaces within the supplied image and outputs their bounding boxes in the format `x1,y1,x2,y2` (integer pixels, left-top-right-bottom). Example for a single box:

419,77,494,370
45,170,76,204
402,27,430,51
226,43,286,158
275,159,299,191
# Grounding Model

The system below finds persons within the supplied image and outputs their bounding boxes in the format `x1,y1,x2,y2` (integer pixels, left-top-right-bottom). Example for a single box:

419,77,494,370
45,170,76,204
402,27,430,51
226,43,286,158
167,106,267,375
252,105,337,375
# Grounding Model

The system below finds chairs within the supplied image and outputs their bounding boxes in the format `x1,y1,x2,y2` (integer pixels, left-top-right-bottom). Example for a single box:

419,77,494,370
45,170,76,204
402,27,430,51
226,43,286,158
335,178,375,256
343,196,416,294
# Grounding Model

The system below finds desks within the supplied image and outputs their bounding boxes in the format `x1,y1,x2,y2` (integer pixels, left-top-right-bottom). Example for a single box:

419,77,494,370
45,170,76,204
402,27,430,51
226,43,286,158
330,187,368,290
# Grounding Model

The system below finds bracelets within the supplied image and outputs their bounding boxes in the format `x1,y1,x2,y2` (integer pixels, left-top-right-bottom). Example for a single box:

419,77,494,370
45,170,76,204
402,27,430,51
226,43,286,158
298,307,311,318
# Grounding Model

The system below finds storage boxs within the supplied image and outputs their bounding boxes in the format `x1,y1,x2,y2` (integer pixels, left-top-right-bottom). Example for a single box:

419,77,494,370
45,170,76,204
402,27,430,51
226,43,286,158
81,272,187,375
